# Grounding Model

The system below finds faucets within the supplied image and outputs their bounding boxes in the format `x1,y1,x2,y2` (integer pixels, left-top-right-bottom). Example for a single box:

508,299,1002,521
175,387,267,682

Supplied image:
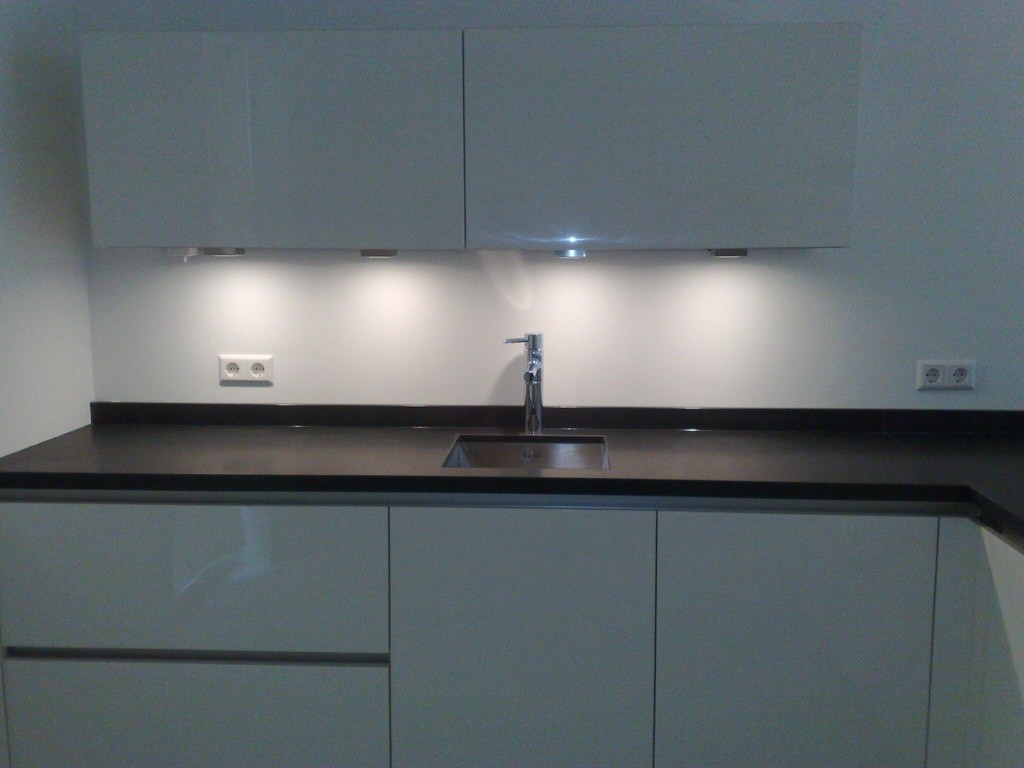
503,331,544,436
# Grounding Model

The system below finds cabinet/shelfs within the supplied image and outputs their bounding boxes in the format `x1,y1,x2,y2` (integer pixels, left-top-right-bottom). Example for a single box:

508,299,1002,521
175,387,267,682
0,500,388,767
390,506,939,768
79,22,858,250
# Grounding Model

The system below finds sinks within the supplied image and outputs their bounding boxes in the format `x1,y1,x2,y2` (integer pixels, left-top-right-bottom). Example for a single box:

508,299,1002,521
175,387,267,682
440,433,613,471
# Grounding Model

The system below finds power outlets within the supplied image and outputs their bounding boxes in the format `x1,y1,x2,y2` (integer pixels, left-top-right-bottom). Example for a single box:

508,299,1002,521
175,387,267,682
218,353,274,385
916,359,976,389
